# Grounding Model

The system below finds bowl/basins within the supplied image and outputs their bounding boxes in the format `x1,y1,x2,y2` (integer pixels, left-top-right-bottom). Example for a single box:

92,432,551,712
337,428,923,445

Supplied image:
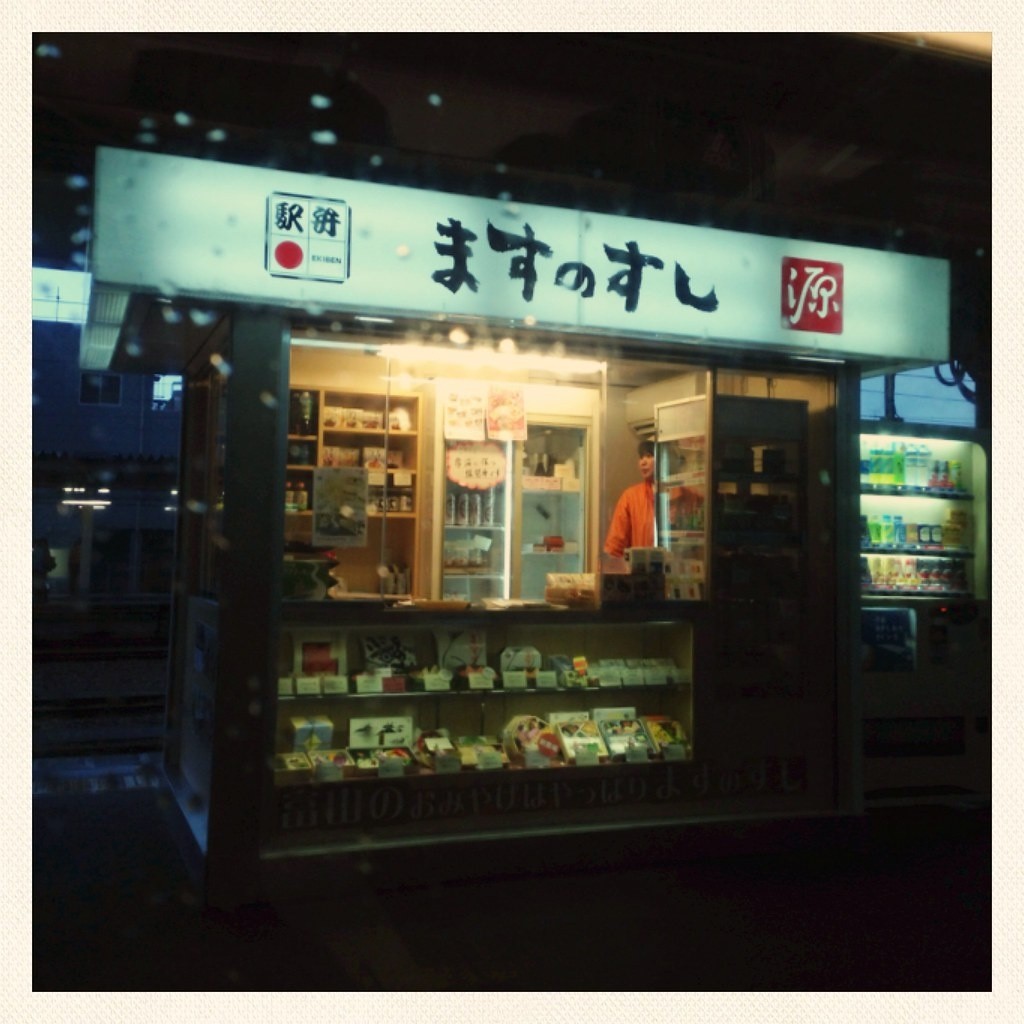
444,548,469,567
469,548,499,569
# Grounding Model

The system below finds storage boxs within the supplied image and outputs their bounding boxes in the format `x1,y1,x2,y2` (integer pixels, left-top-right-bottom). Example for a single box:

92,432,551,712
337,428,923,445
289,716,333,751
599,717,657,761
554,720,608,764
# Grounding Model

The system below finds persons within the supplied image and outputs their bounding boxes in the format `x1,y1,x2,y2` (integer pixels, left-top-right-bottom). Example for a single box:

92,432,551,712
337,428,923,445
603,441,703,559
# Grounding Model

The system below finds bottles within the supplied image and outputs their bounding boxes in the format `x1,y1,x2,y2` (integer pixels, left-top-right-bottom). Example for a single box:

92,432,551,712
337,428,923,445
294,482,308,510
931,460,949,483
285,481,294,504
860,515,871,546
860,440,882,484
457,492,469,524
883,514,893,543
301,443,310,465
870,515,882,543
295,392,314,435
882,444,907,484
469,491,482,526
483,489,494,525
445,492,456,525
906,442,930,486
893,514,906,543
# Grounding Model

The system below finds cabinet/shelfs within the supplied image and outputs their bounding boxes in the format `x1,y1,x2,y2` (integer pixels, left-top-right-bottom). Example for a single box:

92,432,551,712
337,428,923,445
269,607,689,793
285,383,423,599
